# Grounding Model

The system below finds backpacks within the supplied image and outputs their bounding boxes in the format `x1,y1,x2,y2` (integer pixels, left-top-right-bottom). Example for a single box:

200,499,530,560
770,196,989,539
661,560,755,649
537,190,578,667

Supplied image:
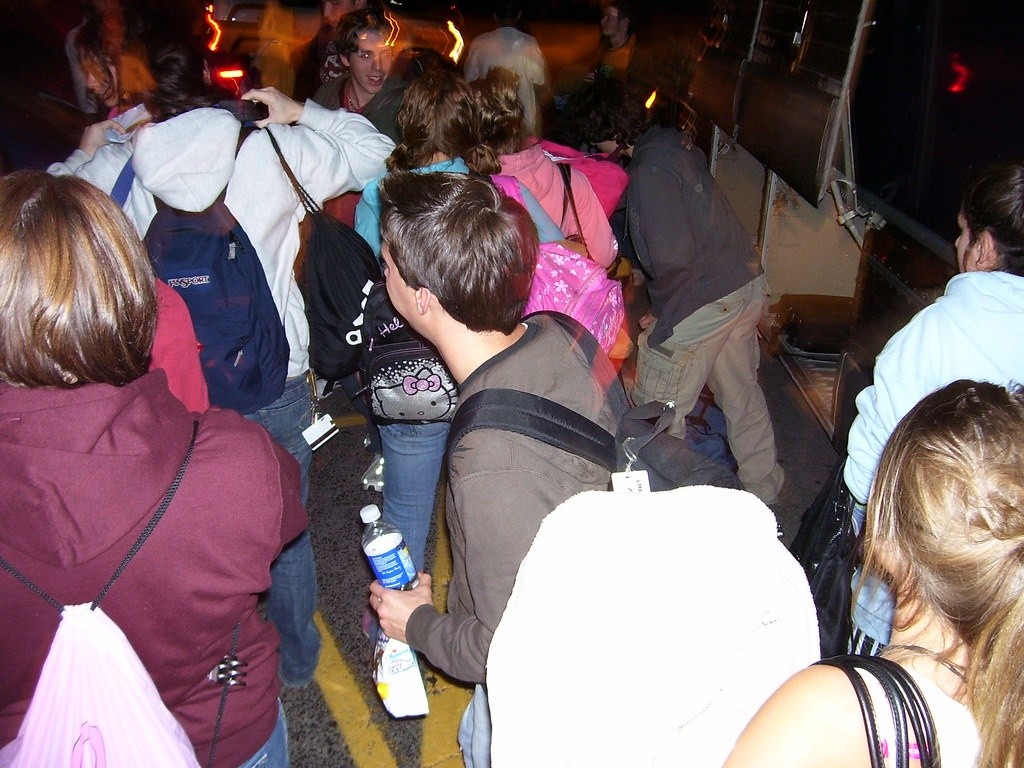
360,275,462,425
448,312,742,491
0,407,201,768
491,171,625,359
137,124,288,415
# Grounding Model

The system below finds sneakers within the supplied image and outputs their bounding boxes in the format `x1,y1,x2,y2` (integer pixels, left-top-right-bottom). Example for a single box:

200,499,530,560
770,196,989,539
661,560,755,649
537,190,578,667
362,455,384,492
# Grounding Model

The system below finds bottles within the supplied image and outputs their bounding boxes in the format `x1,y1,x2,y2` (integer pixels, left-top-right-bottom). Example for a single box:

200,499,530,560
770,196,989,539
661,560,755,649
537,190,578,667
359,504,421,596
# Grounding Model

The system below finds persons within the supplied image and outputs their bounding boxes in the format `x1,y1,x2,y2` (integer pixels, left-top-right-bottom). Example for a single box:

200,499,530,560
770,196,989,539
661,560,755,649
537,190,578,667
0,171,309,768
0,0,1024,768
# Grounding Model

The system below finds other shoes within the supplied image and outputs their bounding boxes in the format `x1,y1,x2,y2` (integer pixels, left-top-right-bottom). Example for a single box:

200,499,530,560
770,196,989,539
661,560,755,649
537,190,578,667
362,602,375,639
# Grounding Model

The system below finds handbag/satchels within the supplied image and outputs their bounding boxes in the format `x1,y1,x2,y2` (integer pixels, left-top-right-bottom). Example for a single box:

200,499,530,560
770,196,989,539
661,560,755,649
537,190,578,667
789,455,869,660
260,124,389,377
678,394,735,473
